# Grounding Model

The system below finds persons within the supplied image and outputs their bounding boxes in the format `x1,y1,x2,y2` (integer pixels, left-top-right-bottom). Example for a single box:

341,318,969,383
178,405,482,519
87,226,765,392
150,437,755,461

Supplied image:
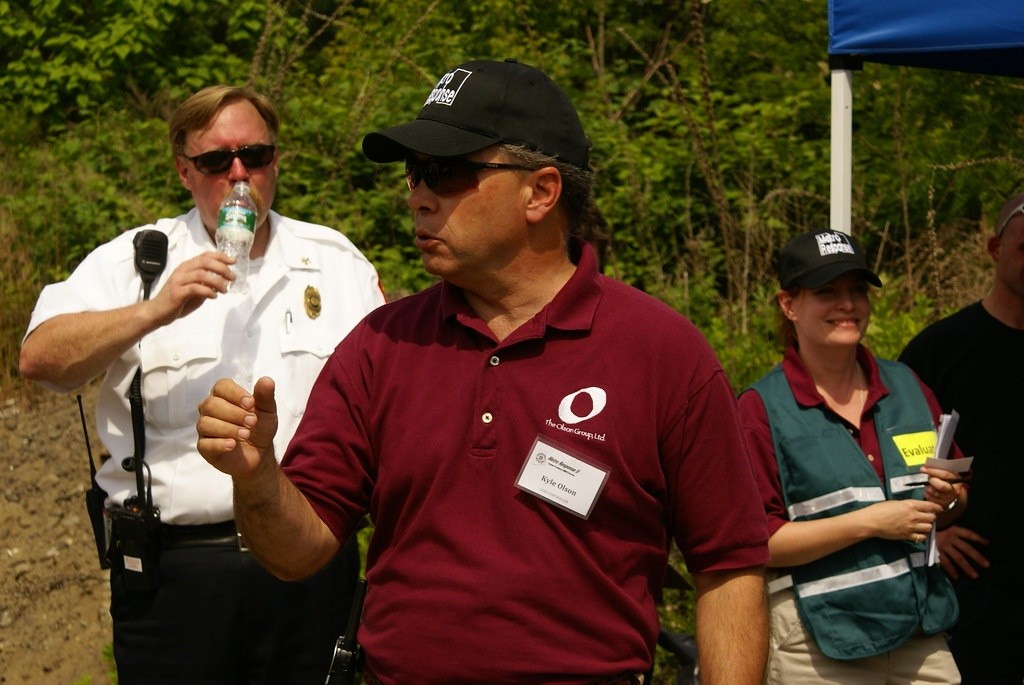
197,61,775,685
736,229,972,685
17,86,388,685
900,189,1024,685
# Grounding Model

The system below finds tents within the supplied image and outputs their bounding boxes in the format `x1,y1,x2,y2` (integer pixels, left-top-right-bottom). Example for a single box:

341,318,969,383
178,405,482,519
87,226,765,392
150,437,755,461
828,0,1024,245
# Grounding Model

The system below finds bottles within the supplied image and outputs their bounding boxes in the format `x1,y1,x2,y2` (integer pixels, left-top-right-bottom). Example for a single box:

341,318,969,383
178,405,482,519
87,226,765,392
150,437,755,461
215,182,258,297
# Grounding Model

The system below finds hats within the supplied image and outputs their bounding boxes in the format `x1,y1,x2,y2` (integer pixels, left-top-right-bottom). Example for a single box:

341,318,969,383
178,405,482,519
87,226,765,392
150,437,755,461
363,58,589,170
778,228,882,289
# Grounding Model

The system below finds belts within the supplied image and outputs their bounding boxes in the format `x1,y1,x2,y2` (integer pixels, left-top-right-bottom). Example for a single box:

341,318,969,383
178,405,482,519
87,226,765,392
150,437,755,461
151,533,252,552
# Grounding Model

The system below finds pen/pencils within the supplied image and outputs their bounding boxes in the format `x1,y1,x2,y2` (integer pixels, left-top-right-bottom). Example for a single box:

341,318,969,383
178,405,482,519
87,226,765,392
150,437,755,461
901,477,973,488
285,305,294,336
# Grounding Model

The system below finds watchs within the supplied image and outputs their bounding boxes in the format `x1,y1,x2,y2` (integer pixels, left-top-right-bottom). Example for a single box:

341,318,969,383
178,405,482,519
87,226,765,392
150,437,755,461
943,496,958,511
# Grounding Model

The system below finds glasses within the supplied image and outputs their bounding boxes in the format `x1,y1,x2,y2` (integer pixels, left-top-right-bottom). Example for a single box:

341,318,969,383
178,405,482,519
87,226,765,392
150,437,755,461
183,145,276,175
406,156,535,195
998,202,1024,238
